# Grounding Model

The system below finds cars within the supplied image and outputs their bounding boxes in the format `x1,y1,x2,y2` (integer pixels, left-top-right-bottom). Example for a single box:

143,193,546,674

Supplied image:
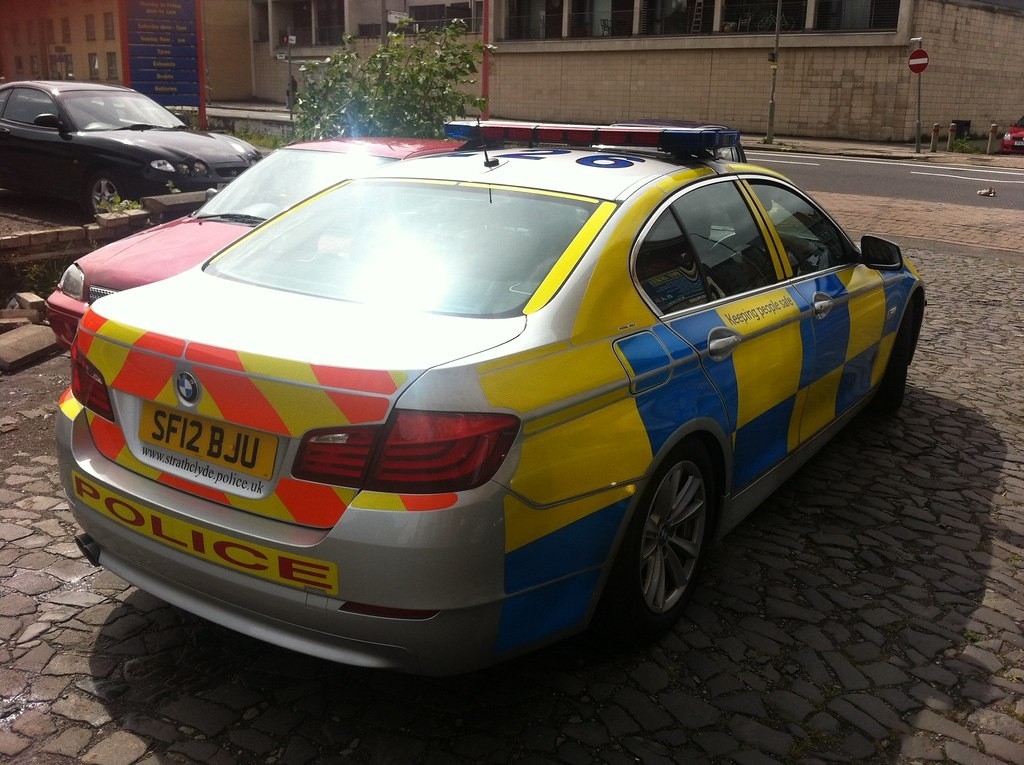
43,139,472,363
54,104,922,680
999,116,1024,155
1,77,268,223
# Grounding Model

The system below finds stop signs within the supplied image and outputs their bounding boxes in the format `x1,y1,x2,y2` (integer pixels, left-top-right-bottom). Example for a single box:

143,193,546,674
907,47,929,74
